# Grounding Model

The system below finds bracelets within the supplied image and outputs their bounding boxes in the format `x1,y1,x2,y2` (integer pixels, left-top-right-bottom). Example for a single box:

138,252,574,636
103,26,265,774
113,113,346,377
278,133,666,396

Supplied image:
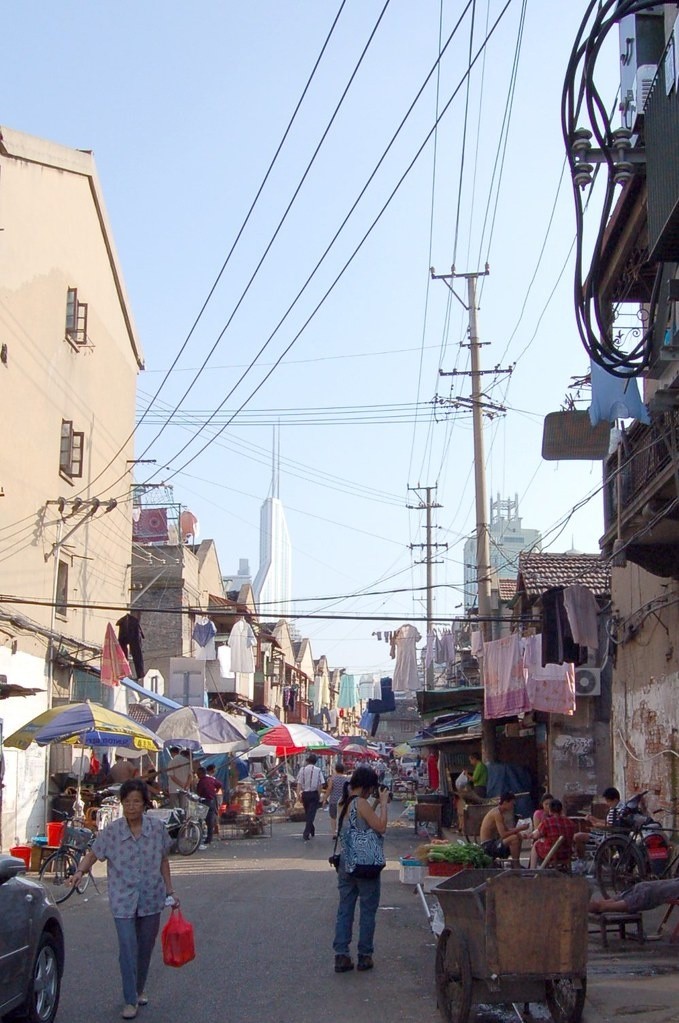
77,870,84,875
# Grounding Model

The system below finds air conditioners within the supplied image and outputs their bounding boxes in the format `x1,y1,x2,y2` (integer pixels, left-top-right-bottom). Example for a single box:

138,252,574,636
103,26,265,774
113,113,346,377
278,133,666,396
576,668,601,697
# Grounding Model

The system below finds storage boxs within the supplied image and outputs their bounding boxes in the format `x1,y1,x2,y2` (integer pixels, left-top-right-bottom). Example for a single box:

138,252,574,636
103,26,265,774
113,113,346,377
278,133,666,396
399,856,427,867
429,861,474,877
31,846,52,872
399,866,429,885
424,876,450,895
147,808,171,824
31,836,48,846
51,851,65,873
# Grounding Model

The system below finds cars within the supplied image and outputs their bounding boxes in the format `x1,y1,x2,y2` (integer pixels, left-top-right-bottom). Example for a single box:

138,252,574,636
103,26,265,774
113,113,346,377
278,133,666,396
0,853,66,1023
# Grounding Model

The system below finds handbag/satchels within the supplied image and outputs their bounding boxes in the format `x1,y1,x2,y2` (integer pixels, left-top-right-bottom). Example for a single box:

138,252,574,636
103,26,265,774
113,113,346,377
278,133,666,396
455,770,468,792
161,905,196,967
344,797,386,877
329,854,340,873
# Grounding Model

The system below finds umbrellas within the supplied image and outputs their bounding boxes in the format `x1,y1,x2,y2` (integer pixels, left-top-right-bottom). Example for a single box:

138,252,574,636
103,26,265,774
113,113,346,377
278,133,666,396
249,723,340,802
0,699,164,802
144,704,259,775
342,743,378,763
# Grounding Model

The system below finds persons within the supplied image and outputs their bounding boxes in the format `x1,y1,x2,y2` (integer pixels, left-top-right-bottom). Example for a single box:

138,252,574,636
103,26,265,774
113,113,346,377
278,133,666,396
66,778,180,1019
109,755,137,783
180,748,222,844
464,753,488,797
588,878,679,912
523,799,576,870
297,755,325,842
320,764,351,837
167,747,192,810
573,787,628,860
141,769,161,799
377,758,387,780
479,790,529,868
395,766,420,783
532,793,565,830
330,765,389,972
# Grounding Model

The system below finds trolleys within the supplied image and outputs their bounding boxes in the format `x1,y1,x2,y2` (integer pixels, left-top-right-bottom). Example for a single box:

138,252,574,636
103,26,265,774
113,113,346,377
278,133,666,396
414,867,595,1022
414,794,445,839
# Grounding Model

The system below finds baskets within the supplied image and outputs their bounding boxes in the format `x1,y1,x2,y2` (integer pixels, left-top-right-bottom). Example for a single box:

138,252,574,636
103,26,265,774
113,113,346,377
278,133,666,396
189,801,209,819
61,826,91,851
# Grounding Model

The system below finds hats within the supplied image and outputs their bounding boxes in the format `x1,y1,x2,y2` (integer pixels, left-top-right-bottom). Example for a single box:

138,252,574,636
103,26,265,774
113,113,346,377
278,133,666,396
307,755,317,762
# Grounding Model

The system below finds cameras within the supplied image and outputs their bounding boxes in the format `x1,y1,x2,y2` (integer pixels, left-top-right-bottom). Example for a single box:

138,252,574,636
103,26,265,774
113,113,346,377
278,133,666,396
372,785,393,802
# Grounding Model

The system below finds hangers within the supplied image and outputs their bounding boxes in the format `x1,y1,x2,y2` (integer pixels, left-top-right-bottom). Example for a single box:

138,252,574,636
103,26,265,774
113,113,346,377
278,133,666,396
432,624,451,634
198,616,209,625
238,614,247,624
402,617,411,631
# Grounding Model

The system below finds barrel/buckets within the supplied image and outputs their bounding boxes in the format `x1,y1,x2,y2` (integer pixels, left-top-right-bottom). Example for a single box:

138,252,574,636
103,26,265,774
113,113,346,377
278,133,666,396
11,847,31,872
47,823,64,846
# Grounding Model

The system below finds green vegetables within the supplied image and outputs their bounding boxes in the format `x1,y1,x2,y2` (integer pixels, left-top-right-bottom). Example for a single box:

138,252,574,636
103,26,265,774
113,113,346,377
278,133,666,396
417,842,491,870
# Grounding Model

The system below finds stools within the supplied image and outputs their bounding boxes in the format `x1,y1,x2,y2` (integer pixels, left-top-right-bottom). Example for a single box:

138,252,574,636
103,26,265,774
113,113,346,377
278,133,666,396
588,911,644,949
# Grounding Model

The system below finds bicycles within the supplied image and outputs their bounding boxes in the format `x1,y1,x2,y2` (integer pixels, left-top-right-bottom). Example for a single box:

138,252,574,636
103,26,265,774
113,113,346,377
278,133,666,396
258,766,296,816
38,808,102,905
592,787,679,905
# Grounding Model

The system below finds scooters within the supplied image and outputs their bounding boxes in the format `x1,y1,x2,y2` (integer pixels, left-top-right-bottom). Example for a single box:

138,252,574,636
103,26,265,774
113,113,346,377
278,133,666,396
176,786,211,856
618,788,670,883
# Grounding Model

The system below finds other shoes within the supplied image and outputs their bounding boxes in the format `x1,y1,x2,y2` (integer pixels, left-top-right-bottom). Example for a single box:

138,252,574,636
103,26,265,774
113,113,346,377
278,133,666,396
333,835,336,840
311,832,314,836
204,838,212,844
574,863,586,873
358,955,373,970
303,834,310,840
138,991,148,1005
123,1003,138,1018
335,954,353,972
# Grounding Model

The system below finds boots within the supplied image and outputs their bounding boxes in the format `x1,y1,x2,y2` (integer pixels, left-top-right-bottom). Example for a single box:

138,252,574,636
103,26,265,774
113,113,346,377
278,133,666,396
213,824,219,834
